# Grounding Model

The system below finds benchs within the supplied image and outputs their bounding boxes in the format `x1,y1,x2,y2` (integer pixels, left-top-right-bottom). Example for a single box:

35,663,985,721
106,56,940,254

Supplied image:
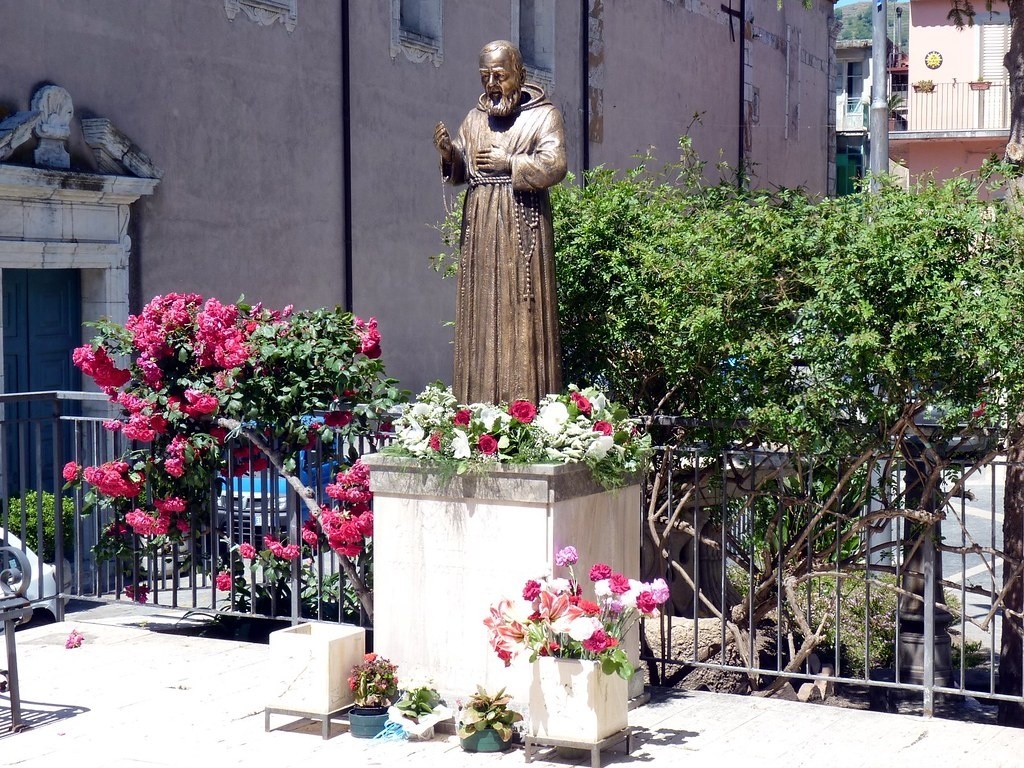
1,547,35,729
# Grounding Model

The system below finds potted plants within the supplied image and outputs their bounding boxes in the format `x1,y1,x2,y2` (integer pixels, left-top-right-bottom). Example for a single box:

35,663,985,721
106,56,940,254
457,683,524,752
394,686,445,740
347,652,401,715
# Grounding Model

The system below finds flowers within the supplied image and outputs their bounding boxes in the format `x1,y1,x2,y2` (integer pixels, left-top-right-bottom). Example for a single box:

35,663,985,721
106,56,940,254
483,545,673,680
385,377,654,494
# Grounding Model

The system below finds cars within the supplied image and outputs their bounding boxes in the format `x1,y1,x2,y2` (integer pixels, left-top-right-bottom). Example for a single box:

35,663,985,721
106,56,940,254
137,528,194,576
0,528,73,638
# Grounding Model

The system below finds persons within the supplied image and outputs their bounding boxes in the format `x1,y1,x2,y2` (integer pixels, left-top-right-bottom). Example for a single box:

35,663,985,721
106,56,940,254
434,41,570,406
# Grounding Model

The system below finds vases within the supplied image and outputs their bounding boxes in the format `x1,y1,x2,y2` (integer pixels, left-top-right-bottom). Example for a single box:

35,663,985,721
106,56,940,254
513,655,629,742
267,622,367,714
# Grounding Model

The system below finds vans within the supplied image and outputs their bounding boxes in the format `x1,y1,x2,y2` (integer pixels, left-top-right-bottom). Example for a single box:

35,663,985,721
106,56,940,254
215,416,341,555
